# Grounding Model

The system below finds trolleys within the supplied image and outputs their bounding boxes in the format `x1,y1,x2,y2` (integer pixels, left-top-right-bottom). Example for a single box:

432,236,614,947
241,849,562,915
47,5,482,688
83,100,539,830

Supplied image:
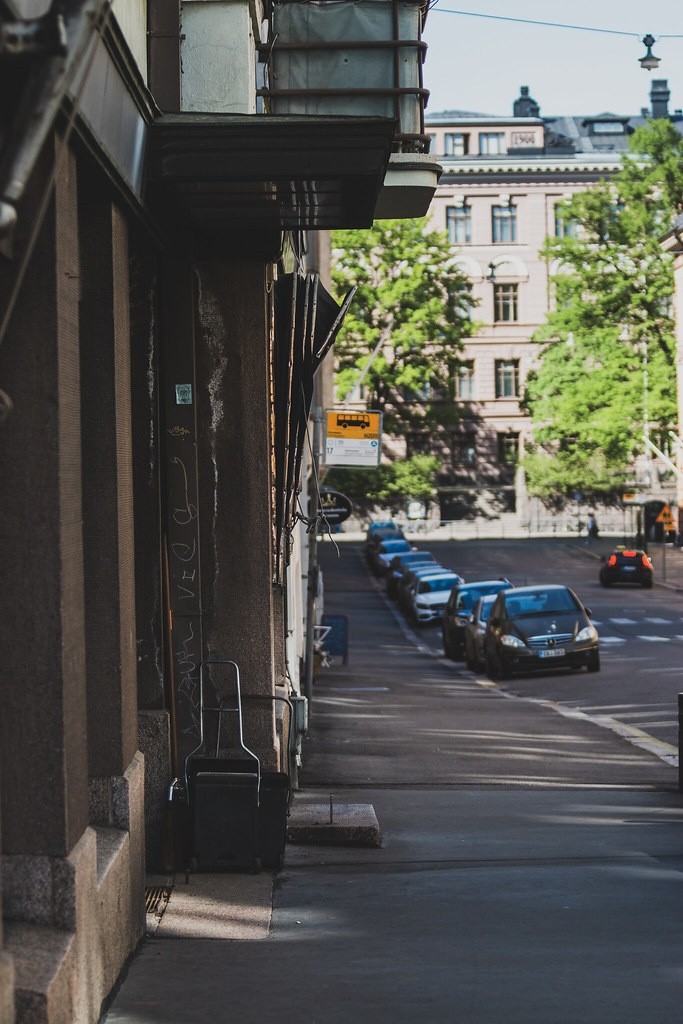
166,656,296,875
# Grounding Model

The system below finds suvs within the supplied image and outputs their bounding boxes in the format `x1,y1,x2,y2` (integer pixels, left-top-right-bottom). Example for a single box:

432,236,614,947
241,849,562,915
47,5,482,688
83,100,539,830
440,576,519,663
479,581,602,679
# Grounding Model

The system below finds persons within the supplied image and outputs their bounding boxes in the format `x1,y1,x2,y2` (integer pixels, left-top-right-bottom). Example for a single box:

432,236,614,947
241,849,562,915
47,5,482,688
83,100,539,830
585,513,602,546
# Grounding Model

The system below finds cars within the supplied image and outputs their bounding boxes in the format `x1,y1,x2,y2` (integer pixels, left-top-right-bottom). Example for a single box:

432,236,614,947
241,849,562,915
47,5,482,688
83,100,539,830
454,594,499,671
362,519,470,624
599,544,655,589
